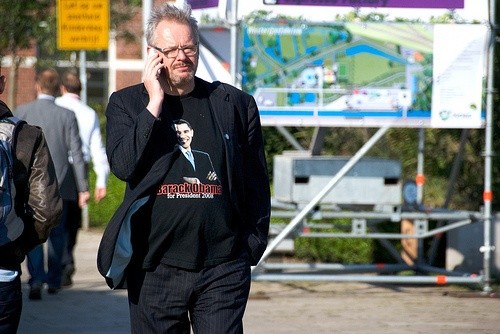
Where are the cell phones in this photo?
[149,48,162,74]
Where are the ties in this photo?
[186,151,195,172]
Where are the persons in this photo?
[0,66,111,334]
[96,6,271,334]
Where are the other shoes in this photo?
[63,262,76,286]
[29,286,42,299]
[48,288,56,294]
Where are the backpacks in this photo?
[0,116,27,246]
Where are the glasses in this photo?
[148,43,198,59]
